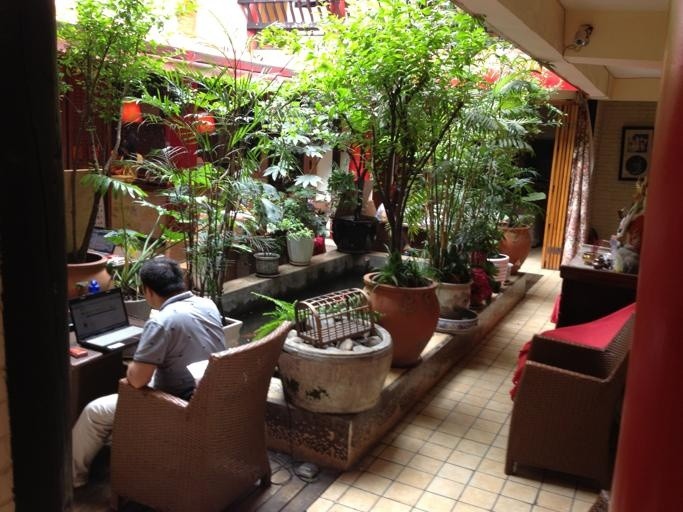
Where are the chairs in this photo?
[358,200,392,256]
[109,321,292,512]
[504,312,636,489]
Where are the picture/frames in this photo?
[618,124,653,180]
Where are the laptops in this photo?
[69,286,144,351]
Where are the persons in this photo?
[71,256,227,499]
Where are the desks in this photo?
[64,320,146,512]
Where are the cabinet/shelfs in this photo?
[555,243,638,327]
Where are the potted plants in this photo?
[249,290,393,414]
[124,6,307,349]
[54,0,199,307]
[159,190,276,296]
[500,71,567,274]
[307,17,379,255]
[486,51,532,291]
[253,200,280,278]
[453,225,505,268]
[250,0,499,368]
[286,227,314,265]
[437,304,478,335]
[422,51,558,315]
